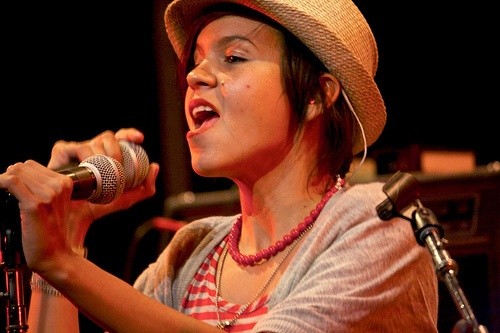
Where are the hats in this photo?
[164,0,386,153]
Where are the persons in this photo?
[0,0,439,333]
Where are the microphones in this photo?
[0,154,127,210]
[63,139,150,192]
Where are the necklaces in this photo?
[215,219,315,331]
[228,177,349,268]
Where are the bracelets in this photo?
[30,275,65,299]
